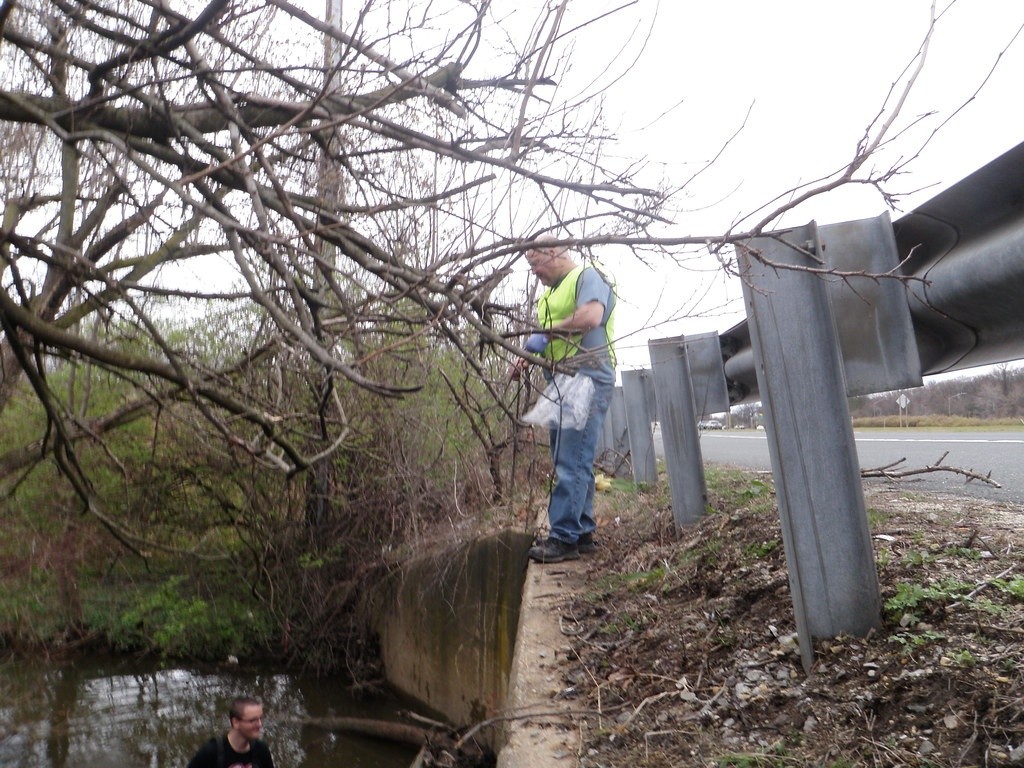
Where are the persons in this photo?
[505,236,618,563]
[185,697,275,768]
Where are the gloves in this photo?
[526,333,548,355]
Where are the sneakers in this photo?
[528,537,580,563]
[577,532,596,553]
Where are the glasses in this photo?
[240,714,263,724]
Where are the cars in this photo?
[735,425,745,429]
[706,420,722,430]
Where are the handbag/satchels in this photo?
[520,373,595,431]
